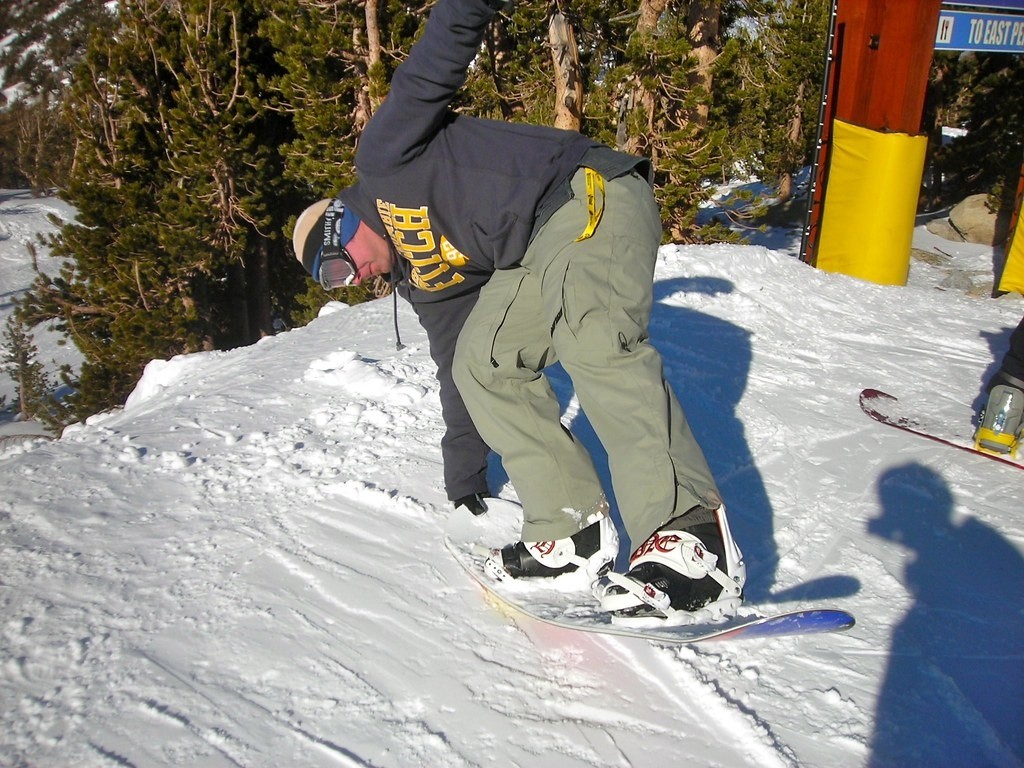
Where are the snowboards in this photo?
[860,388,1024,469]
[444,496,856,644]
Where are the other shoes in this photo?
[976,375,1024,453]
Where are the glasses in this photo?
[318,201,356,290]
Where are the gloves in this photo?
[452,492,488,515]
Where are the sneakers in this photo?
[607,505,743,620]
[486,516,616,584]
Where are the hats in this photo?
[294,198,359,283]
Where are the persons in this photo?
[293,0,748,619]
